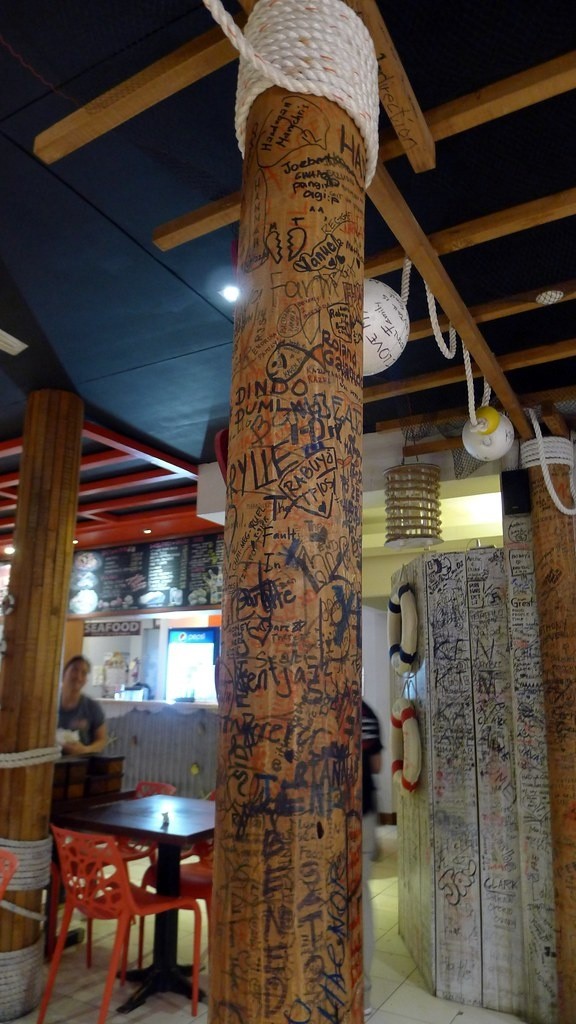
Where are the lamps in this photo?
[383,428,443,550]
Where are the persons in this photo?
[56,654,107,767]
[361,698,383,1016]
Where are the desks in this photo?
[80,795,215,1014]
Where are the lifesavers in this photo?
[385,584,418,674]
[388,697,422,793]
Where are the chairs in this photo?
[36,783,215,1024]
